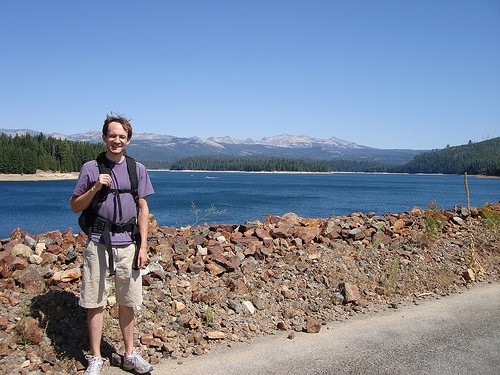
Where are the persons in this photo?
[69,112,155,375]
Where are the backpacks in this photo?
[78,152,142,239]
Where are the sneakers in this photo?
[82,355,106,375]
[124,351,153,374]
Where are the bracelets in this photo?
[139,244,148,249]
